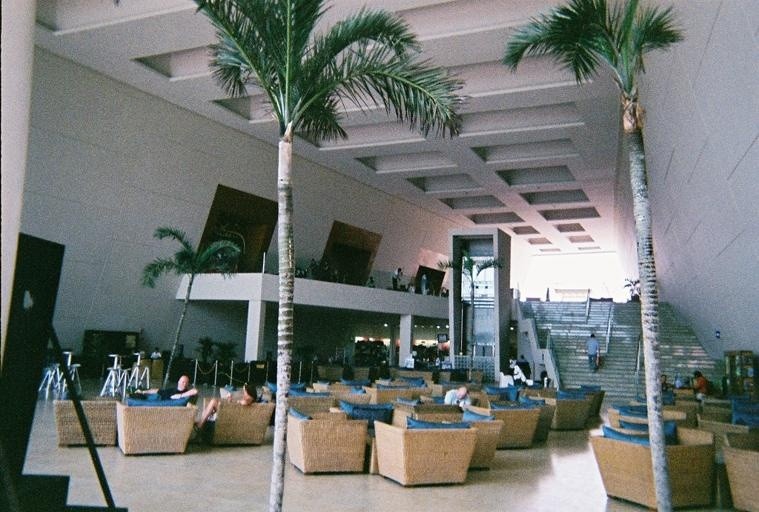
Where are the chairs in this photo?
[52,386,277,457]
[257,364,606,486]
[590,378,759,511]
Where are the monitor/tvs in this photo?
[438,333,448,343]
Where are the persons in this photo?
[343,359,354,382]
[431,366,441,384]
[584,334,600,372]
[661,374,670,391]
[134,374,198,400]
[444,384,470,408]
[150,348,161,360]
[369,361,380,386]
[195,384,258,432]
[498,355,531,388]
[380,361,390,380]
[296,259,449,297]
[691,371,708,394]
